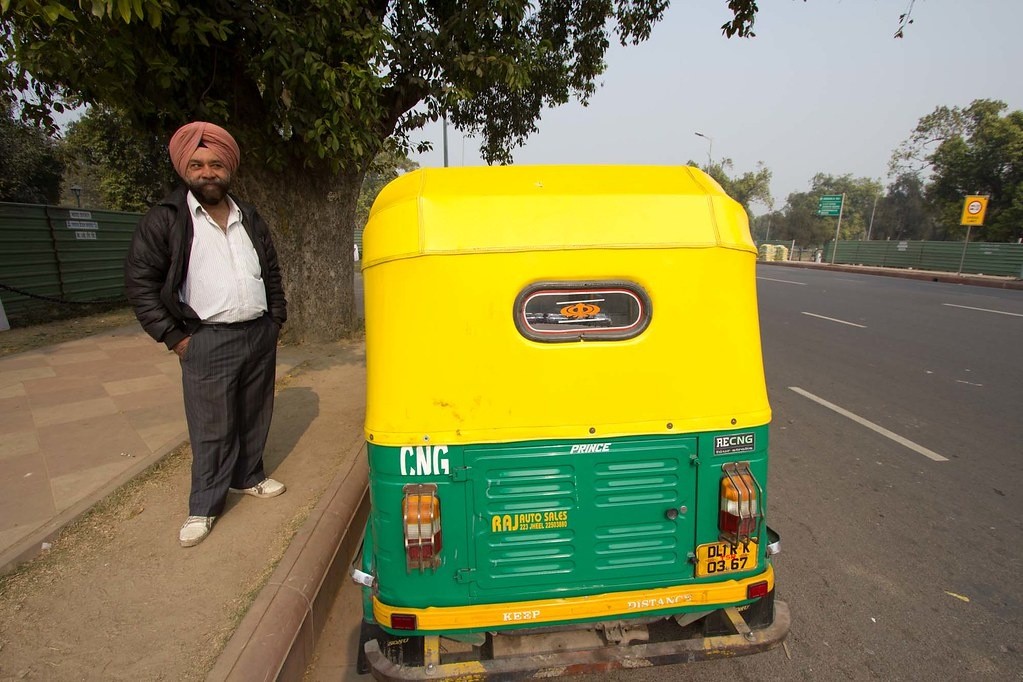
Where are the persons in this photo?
[124,121,288,548]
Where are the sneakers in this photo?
[228,476,286,499]
[178,514,216,547]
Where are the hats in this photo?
[168,122,241,181]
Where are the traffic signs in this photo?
[816,192,845,264]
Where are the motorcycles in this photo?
[351,164,792,682]
